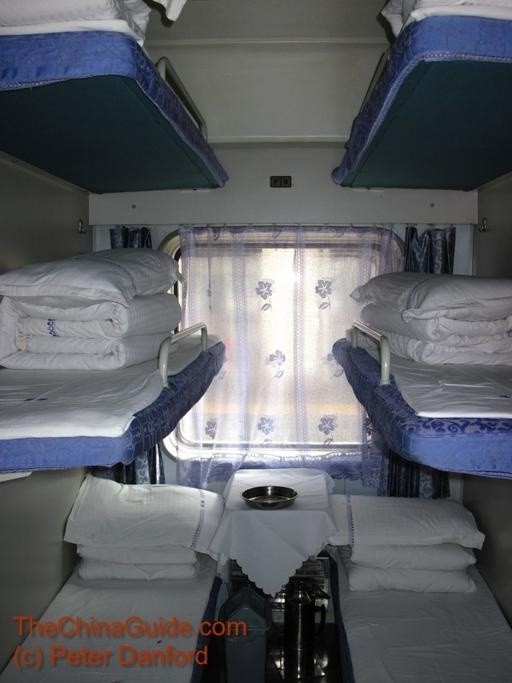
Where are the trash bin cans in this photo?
[218,587,272,683]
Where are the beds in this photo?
[2,29,229,196]
[330,547,512,682]
[330,16,511,191]
[0,322,226,479]
[0,560,224,683]
[333,320,512,481]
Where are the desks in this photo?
[224,468,333,561]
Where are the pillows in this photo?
[61,470,225,560]
[329,494,487,551]
[0,246,183,308]
[348,271,512,325]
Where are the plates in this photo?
[238,485,298,510]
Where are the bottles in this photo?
[284,575,329,652]
